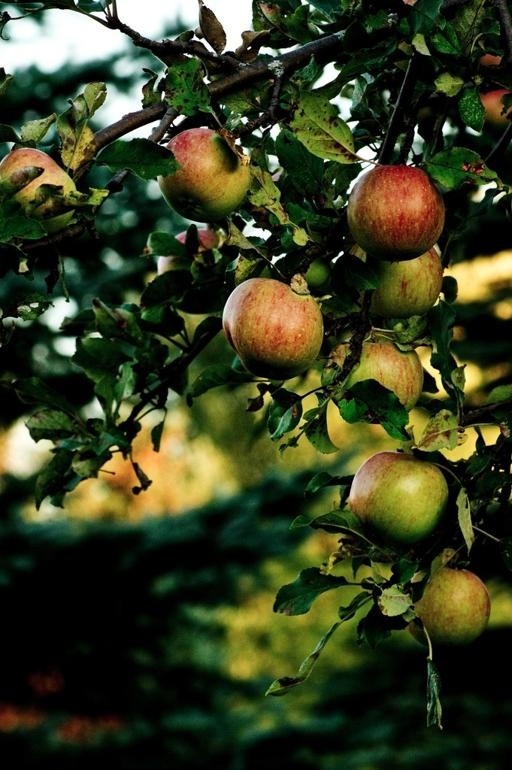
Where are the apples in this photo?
[0,148,77,216]
[408,566,491,649]
[222,277,325,380]
[346,165,446,262]
[349,451,449,546]
[477,89,512,132]
[349,243,443,318]
[157,128,251,222]
[325,328,424,424]
[305,259,333,290]
[480,54,502,67]
[157,228,219,277]
